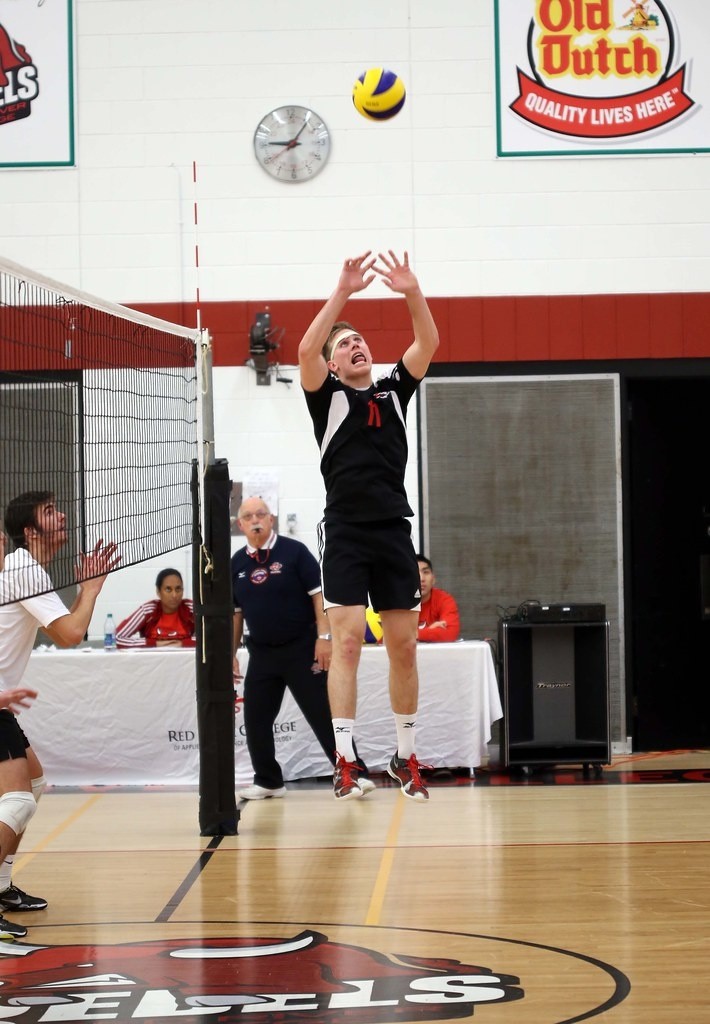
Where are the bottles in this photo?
[104,613,116,653]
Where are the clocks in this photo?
[253,106,331,183]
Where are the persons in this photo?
[419,555,462,641]
[0,491,122,942]
[233,496,378,800]
[299,250,442,802]
[113,567,196,647]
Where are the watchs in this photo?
[318,634,332,641]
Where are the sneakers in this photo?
[239,784,288,799]
[358,778,376,795]
[387,749,430,801]
[0,881,48,911]
[333,751,364,801]
[0,913,28,938]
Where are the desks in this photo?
[10,640,505,787]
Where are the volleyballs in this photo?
[350,66,408,122]
[363,606,384,645]
